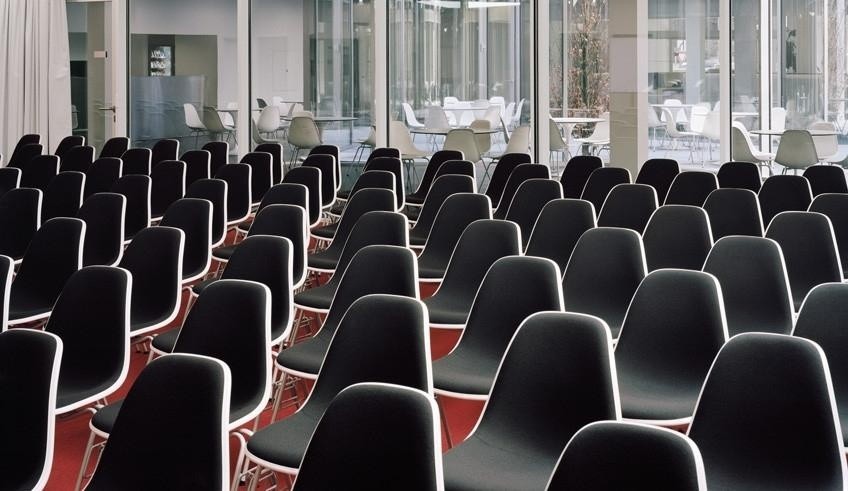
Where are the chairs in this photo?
[549,109,611,177]
[647,98,847,180]
[0,132,847,490]
[184,98,376,176]
[389,93,530,193]
[69,104,80,130]
